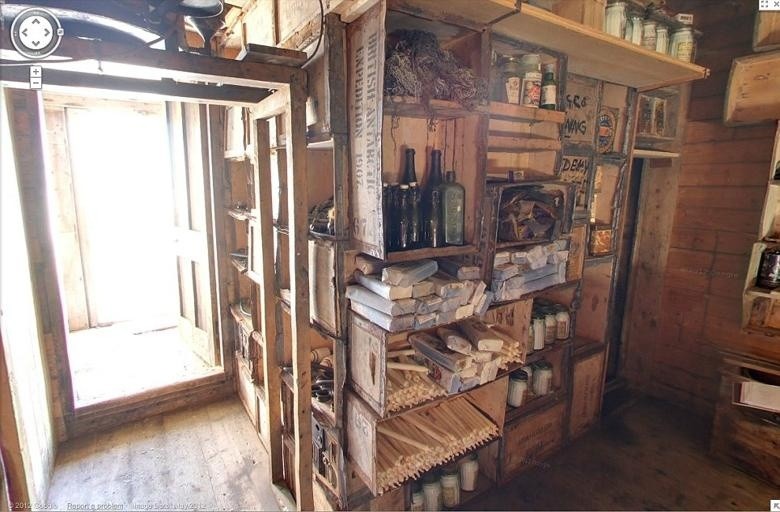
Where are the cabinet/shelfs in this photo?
[223,13,350,511]
[711,118,780,489]
[340,0,712,512]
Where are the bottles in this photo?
[383,182,389,233]
[423,150,445,247]
[668,25,694,62]
[626,16,634,42]
[532,314,546,351]
[556,304,569,340]
[402,150,418,185]
[395,185,410,249]
[424,474,441,512]
[533,361,554,396]
[499,56,522,105]
[392,183,399,203]
[441,466,460,509]
[542,308,557,344]
[459,453,480,492]
[508,370,528,408]
[629,12,643,44]
[641,20,656,51]
[409,183,422,244]
[606,2,628,39]
[520,366,532,390]
[542,65,557,110]
[533,305,540,314]
[410,484,426,512]
[537,297,554,305]
[439,171,465,246]
[656,24,670,54]
[527,322,535,357]
